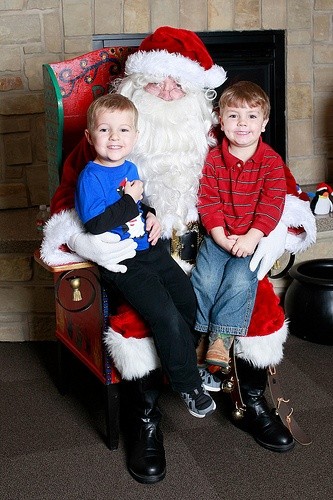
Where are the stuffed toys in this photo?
[310,181,333,215]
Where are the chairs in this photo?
[34,45,295,450]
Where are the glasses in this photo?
[145,88,186,100]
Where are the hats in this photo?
[125,25,228,88]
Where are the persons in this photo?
[75,93,224,419]
[40,25,317,485]
[190,81,287,369]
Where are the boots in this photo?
[231,356,295,452]
[120,368,167,484]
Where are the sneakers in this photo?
[196,364,223,392]
[180,385,216,419]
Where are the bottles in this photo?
[35,205,51,240]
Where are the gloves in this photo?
[66,232,138,273]
[249,220,288,281]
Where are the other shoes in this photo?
[195,333,234,368]
